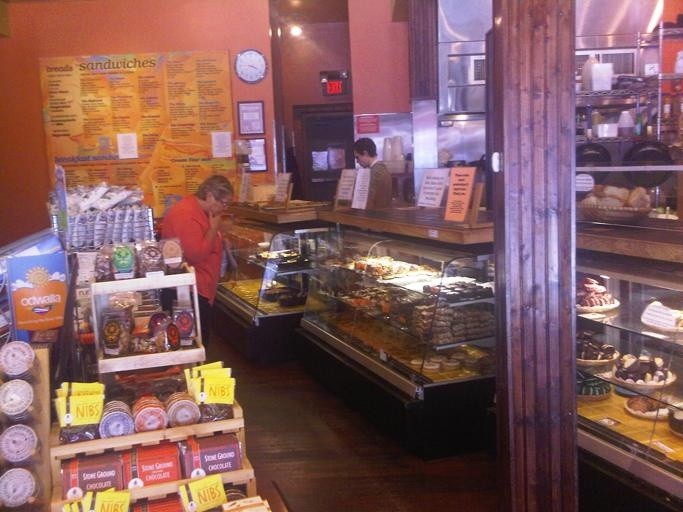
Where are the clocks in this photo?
[234,49,268,84]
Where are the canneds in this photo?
[0,340,41,511]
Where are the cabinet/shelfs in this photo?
[574,25,682,208]
[293,229,494,458]
[212,202,343,367]
[2,198,273,512]
[574,206,683,506]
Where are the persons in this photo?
[160,174,234,349]
[351,137,395,214]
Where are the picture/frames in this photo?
[242,138,268,172]
[237,100,265,134]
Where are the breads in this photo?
[575,331,682,432]
[641,301,682,333]
[576,274,616,311]
[578,181,653,224]
[306,253,496,378]
